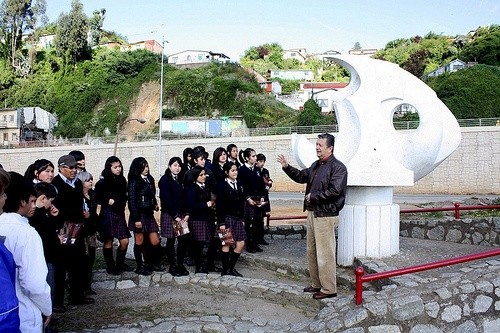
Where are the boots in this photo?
[132,241,165,275]
[117,246,134,271]
[221,251,243,276]
[103,247,122,274]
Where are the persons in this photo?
[277,132,348,299]
[0,143,274,333]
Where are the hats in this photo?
[57,154,82,166]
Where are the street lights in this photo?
[114,118,146,156]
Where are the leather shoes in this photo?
[85,289,97,294]
[70,296,95,303]
[304,286,321,292]
[246,244,263,252]
[53,305,67,312]
[186,256,221,273]
[257,238,269,244]
[313,291,337,298]
[65,279,70,290]
[170,263,189,275]
